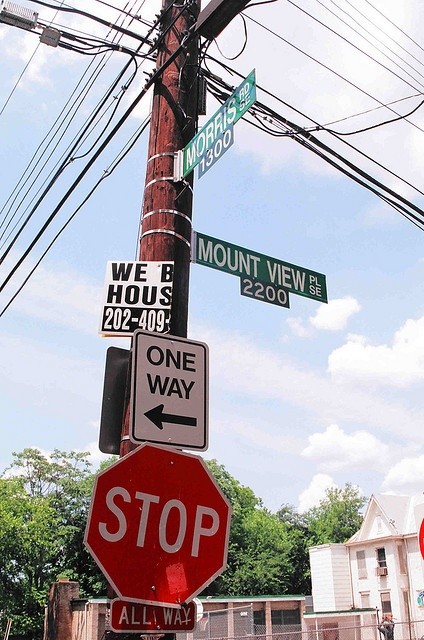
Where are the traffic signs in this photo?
[129,328,210,452]
[176,67,263,190]
[181,226,332,312]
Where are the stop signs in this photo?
[83,440,234,609]
